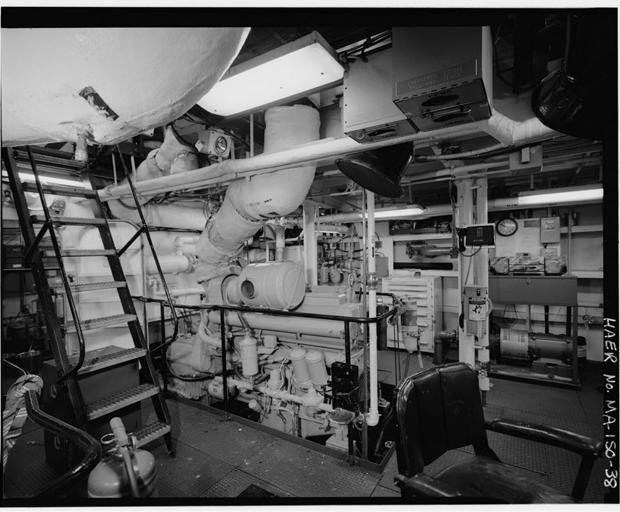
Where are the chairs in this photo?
[394,361,602,504]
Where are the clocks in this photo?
[496,217,517,237]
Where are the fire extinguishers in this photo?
[87,416,160,499]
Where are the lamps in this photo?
[359,204,425,219]
[197,31,346,121]
[517,184,605,206]
